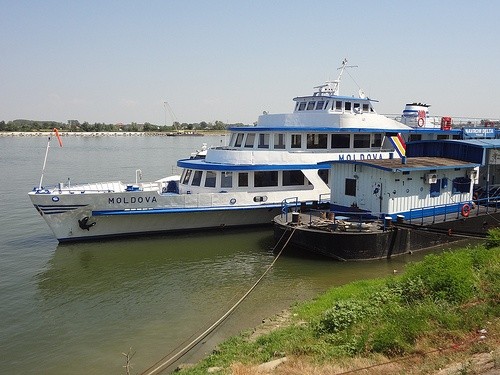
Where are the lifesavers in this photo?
[417,118,424,127]
[461,204,471,216]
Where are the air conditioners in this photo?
[425,173,437,184]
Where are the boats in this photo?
[270,156,500,262]
[27,58,414,245]
